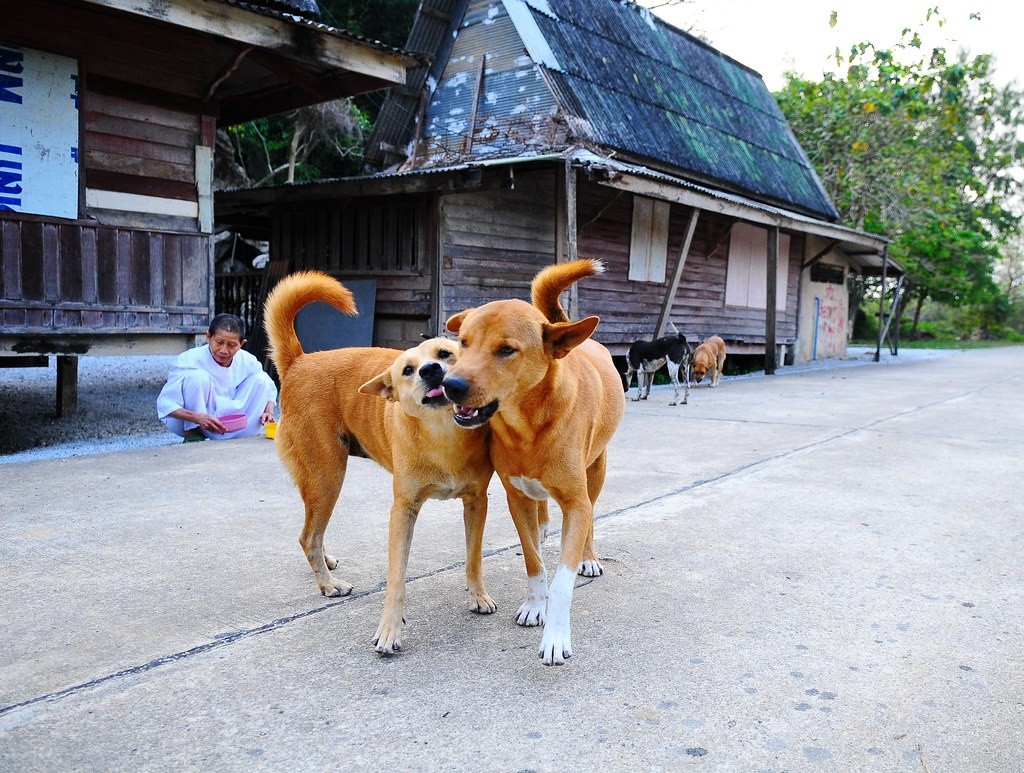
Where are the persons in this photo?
[156,313,278,444]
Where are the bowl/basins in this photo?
[265,423,278,439]
[217,414,246,432]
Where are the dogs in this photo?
[690,334,727,388]
[623,333,692,407]
[442,259,626,666]
[264,270,493,659]
